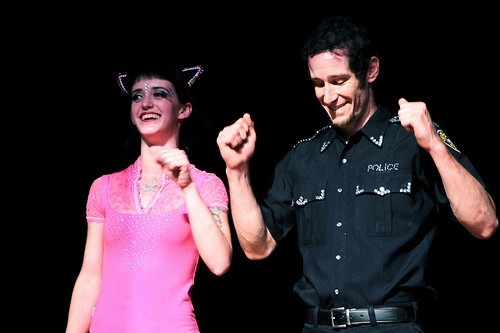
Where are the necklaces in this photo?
[140,182,159,193]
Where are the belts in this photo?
[304,302,422,328]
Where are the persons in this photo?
[216,25,499,333]
[65,56,232,333]
